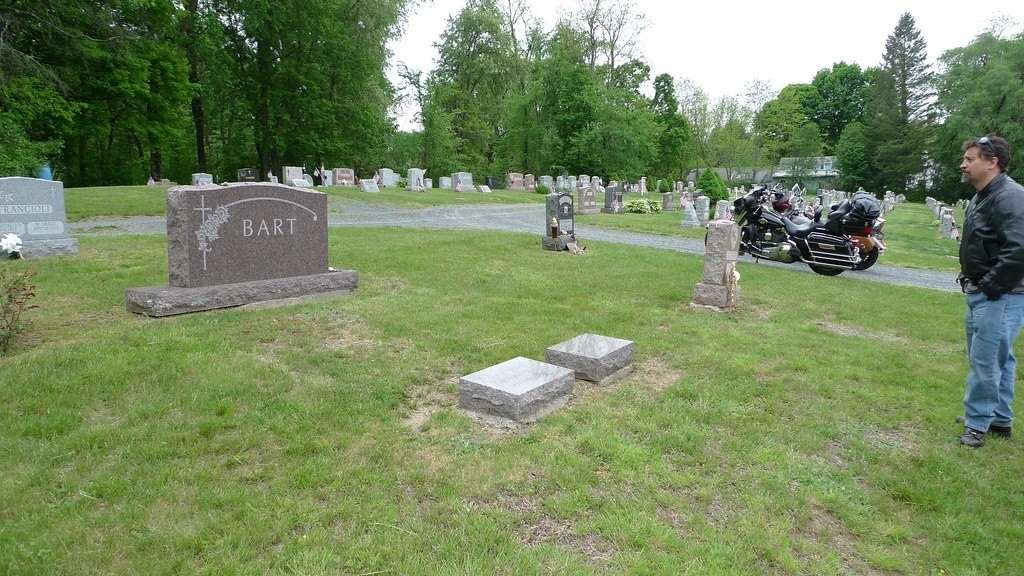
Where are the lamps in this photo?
[550,217,559,243]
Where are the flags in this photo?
[951,225,959,239]
[418,177,423,187]
[314,169,319,177]
[321,164,324,173]
[456,179,464,191]
[373,172,380,181]
[612,194,619,212]
[725,207,732,220]
[681,194,687,207]
[267,170,272,179]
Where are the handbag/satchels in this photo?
[849,191,881,222]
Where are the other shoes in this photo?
[960,428,985,448]
[956,415,1012,438]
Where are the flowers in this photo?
[0,232,23,259]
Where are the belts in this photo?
[961,277,971,284]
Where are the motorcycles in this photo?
[704,173,886,277]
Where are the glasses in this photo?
[979,137,997,154]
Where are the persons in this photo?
[955,136,1024,447]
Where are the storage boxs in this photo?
[839,217,874,237]
